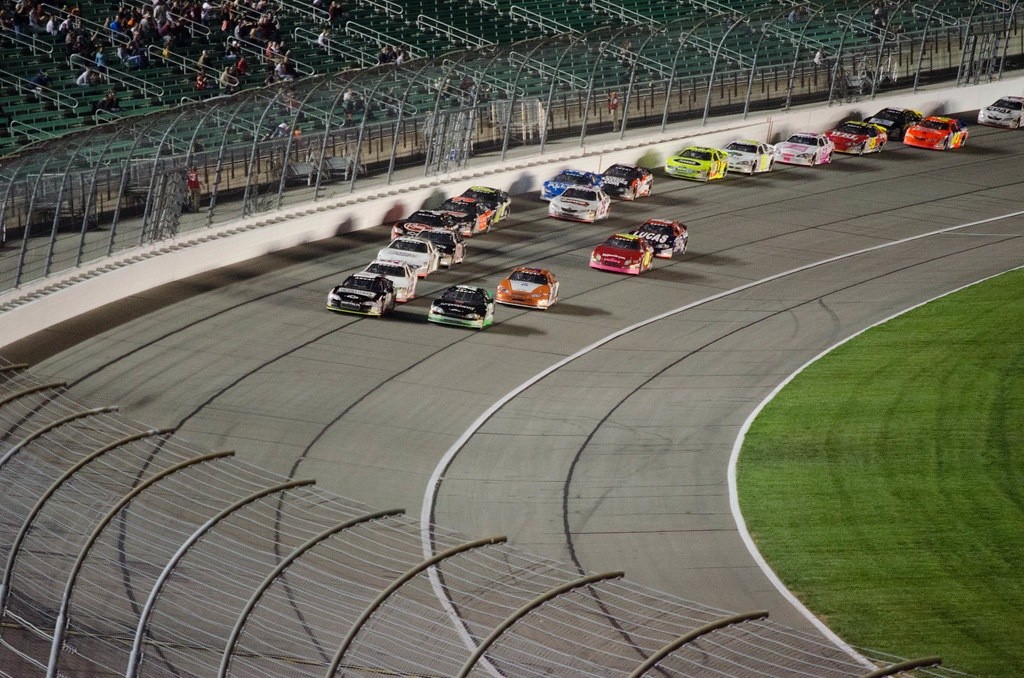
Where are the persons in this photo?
[607,90,620,132]
[868,0,892,43]
[265,116,278,136]
[276,119,290,137]
[94,89,123,115]
[294,124,305,143]
[191,136,205,152]
[788,5,808,25]
[328,42,503,122]
[618,38,664,91]
[720,12,746,33]
[813,44,830,68]
[188,165,205,213]
[375,281,383,292]
[0,0,356,101]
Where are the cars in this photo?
[774,132,835,168]
[722,138,776,175]
[664,146,728,182]
[978,96,1024,130]
[629,218,688,258]
[431,196,494,238]
[589,233,654,275]
[354,259,417,303]
[415,229,466,268]
[428,285,495,330]
[903,116,969,150]
[825,121,888,156]
[390,210,462,242]
[460,187,511,223]
[863,107,924,141]
[599,163,654,200]
[540,168,605,201]
[377,236,440,279]
[496,266,560,311]
[326,276,397,318]
[548,185,611,223]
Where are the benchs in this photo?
[0,0,1024,232]
